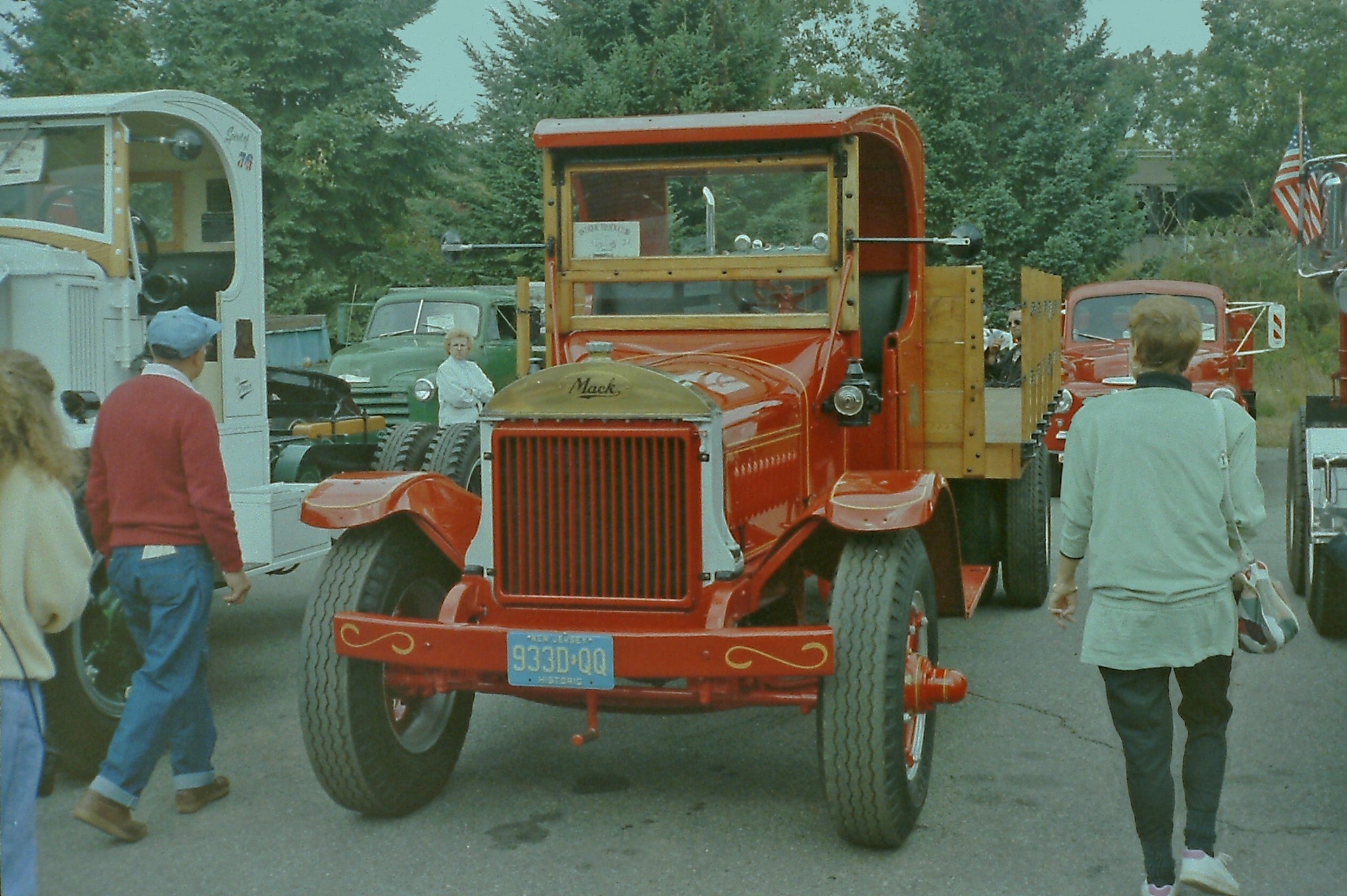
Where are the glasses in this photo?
[1008,319,1021,327]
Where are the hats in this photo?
[145,305,222,359]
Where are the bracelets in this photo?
[1052,584,1078,595]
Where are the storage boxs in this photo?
[228,480,332,566]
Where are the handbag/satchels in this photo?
[36,745,61,798]
[1231,560,1300,656]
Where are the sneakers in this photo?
[1140,878,1174,896]
[72,789,147,842]
[175,776,230,813]
[1178,846,1240,896]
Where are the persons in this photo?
[435,327,495,428]
[984,310,1021,388]
[71,306,252,842]
[0,346,94,896]
[1047,293,1270,895]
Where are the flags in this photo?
[1271,114,1329,249]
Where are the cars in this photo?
[1270,89,1347,638]
[1047,277,1287,499]
[294,99,1065,852]
[1,84,483,783]
[324,289,547,429]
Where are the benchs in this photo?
[587,260,909,403]
[129,252,239,334]
